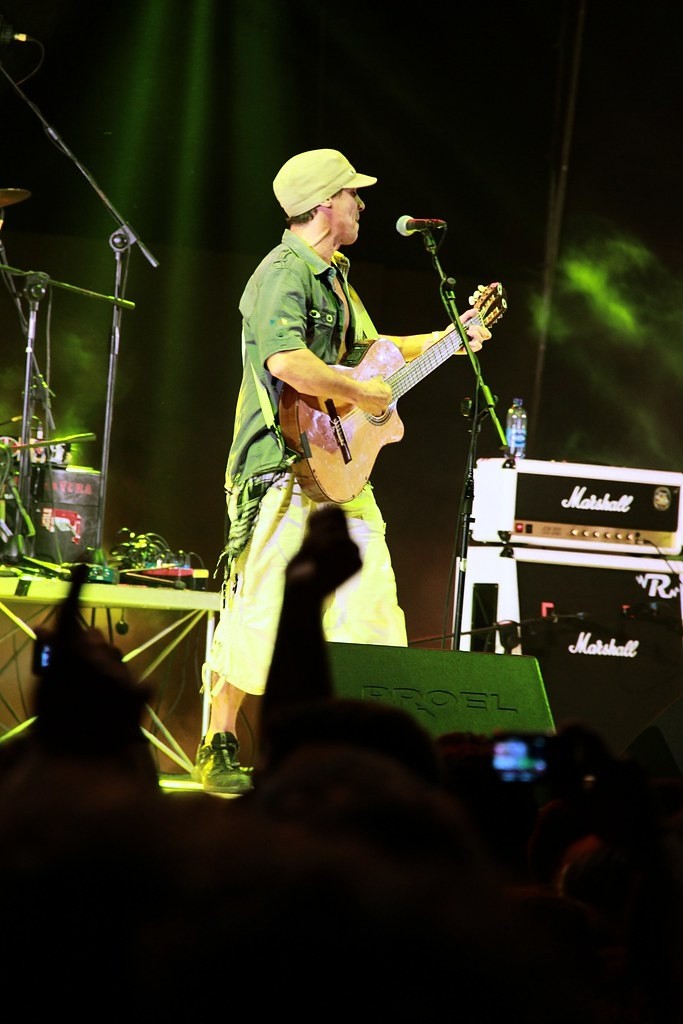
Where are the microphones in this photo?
[0,33,33,43]
[397,215,446,236]
[620,601,665,620]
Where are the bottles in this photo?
[504,398,527,458]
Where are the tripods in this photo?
[0,65,190,590]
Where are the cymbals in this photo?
[0,187,34,207]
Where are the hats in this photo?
[273,149,380,217]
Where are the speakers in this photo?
[325,545,683,781]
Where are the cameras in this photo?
[489,733,549,782]
[31,627,57,675]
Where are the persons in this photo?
[193,148,492,795]
[0,629,683,1024]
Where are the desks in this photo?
[0,574,224,786]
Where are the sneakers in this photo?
[193,731,251,792]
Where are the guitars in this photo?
[279,280,509,506]
